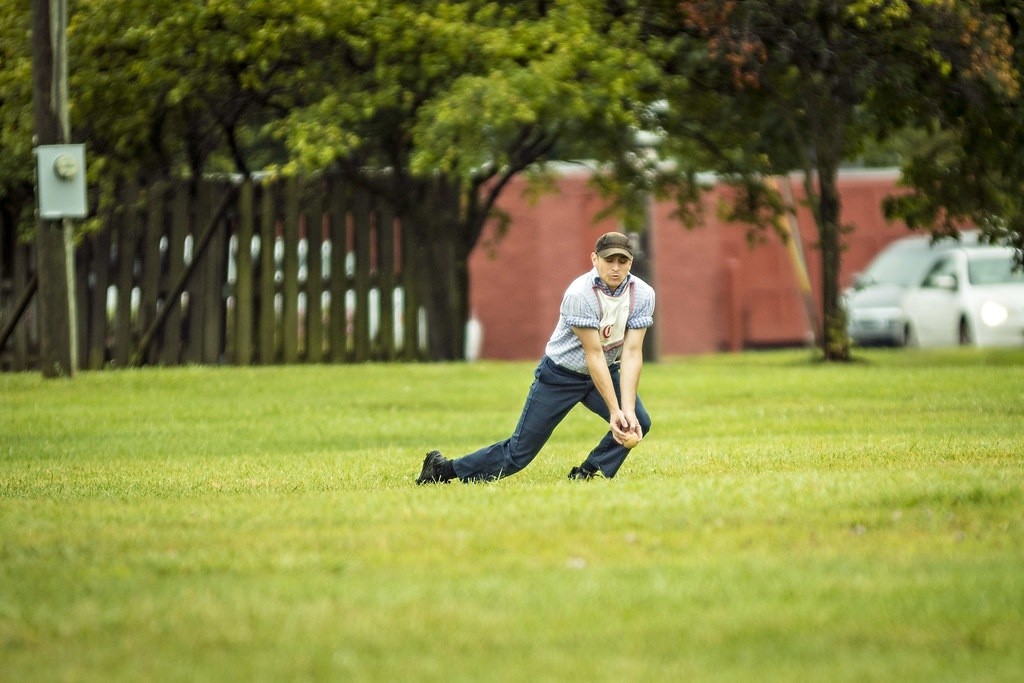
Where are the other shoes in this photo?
[567,466,596,484]
[414,450,452,485]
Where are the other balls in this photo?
[622,430,639,449]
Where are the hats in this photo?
[594,231,634,263]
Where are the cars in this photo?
[843,230,1024,347]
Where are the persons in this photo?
[416,231,655,488]
